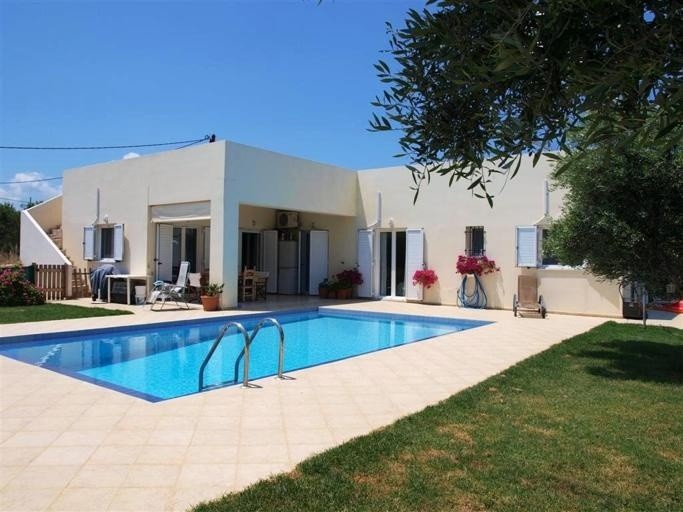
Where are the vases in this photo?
[347,288,353,298]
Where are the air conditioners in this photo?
[276,211,298,229]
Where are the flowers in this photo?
[337,269,363,289]
[455,255,500,276]
[413,269,438,289]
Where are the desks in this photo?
[239,271,269,301]
[106,274,153,305]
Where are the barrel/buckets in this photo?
[135,294,145,305]
[134,286,146,294]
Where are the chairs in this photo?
[512,275,545,318]
[240,265,257,302]
[150,261,191,311]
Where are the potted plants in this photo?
[200,283,225,311]
[319,279,347,298]
